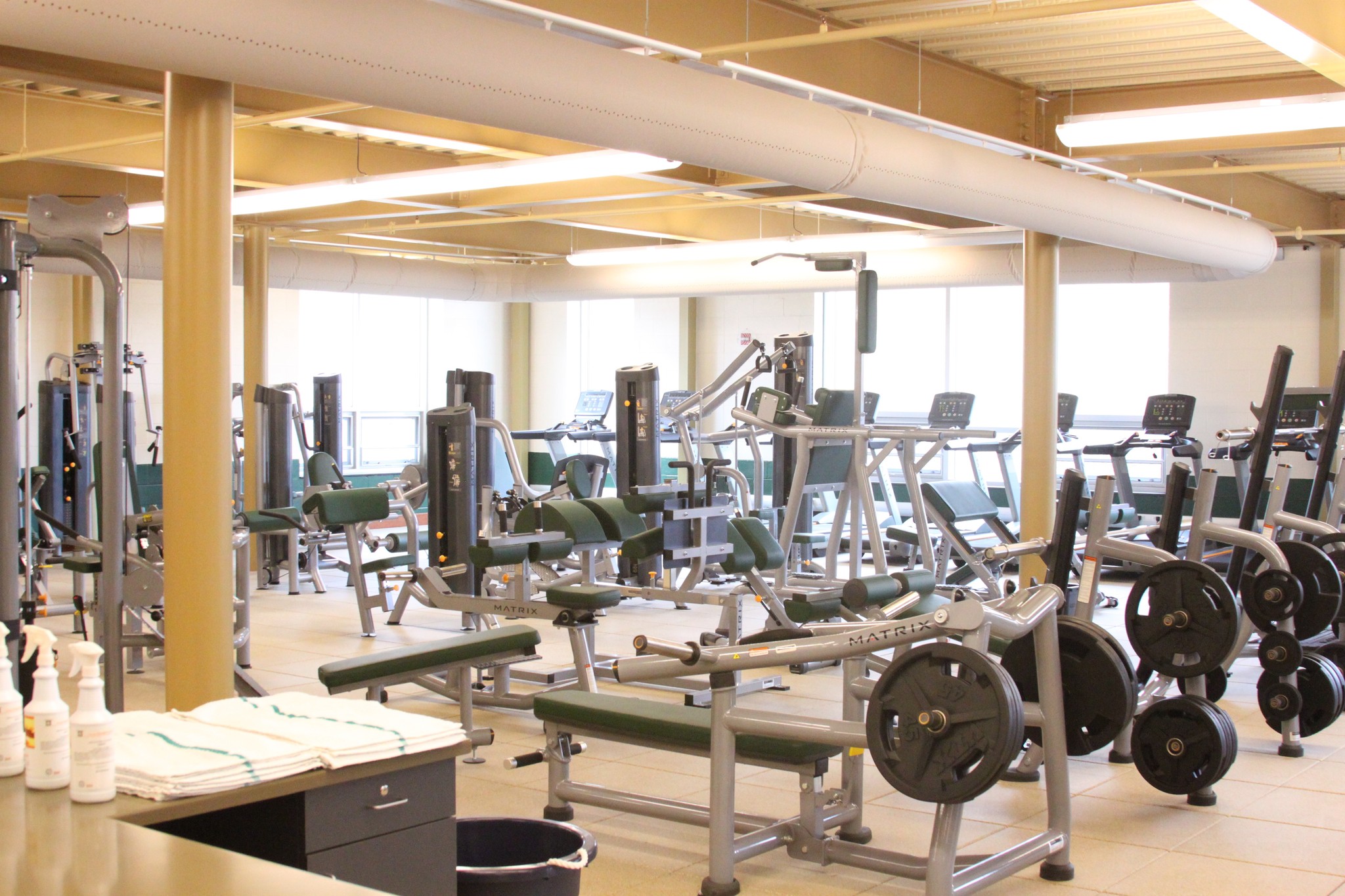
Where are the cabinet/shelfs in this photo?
[301,757,459,896]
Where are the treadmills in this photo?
[543,389,1333,572]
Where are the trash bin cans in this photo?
[456,817,598,896]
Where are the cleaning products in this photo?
[67,640,116,805]
[18,625,70,792]
[0,621,27,779]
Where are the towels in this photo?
[109,690,468,803]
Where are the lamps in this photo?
[1052,8,1345,151]
[127,103,943,272]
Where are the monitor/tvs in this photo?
[1142,394,1195,431]
[574,389,614,419]
[660,390,695,408]
[927,392,975,426]
[1276,410,1319,429]
[1057,393,1078,428]
